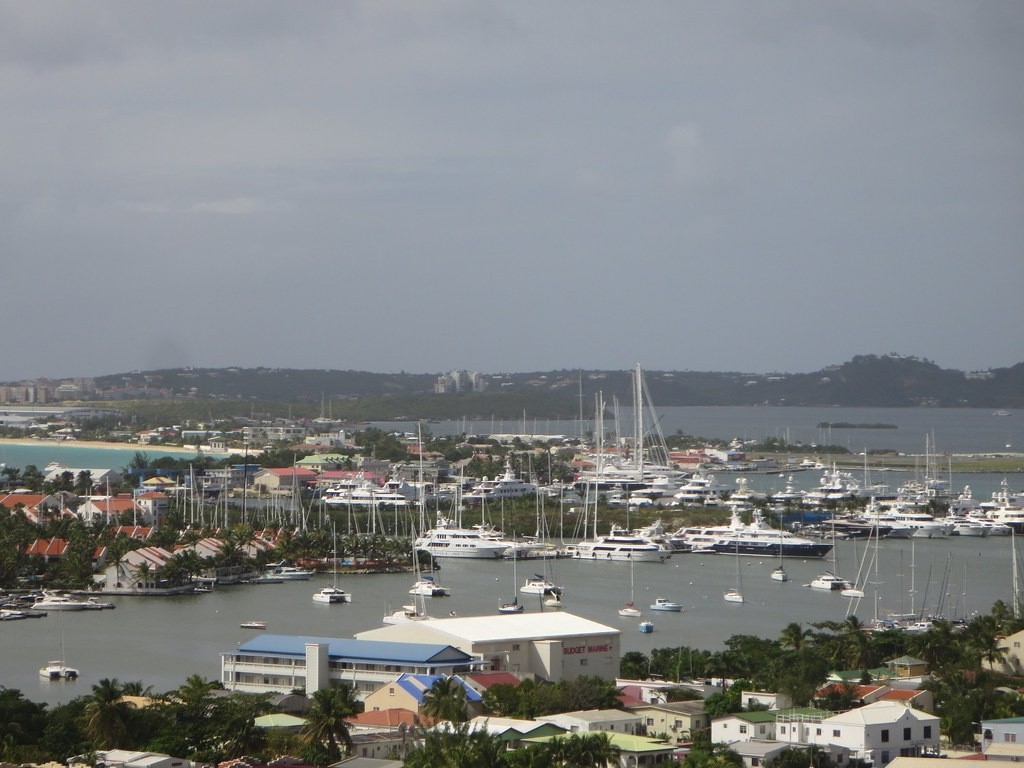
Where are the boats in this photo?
[194,586,212,593]
[641,623,654,633]
[0,588,116,620]
[240,621,267,628]
[243,562,314,583]
[545,598,562,608]
[651,598,683,612]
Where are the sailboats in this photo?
[39,596,79,679]
[312,519,352,604]
[320,360,1024,615]
